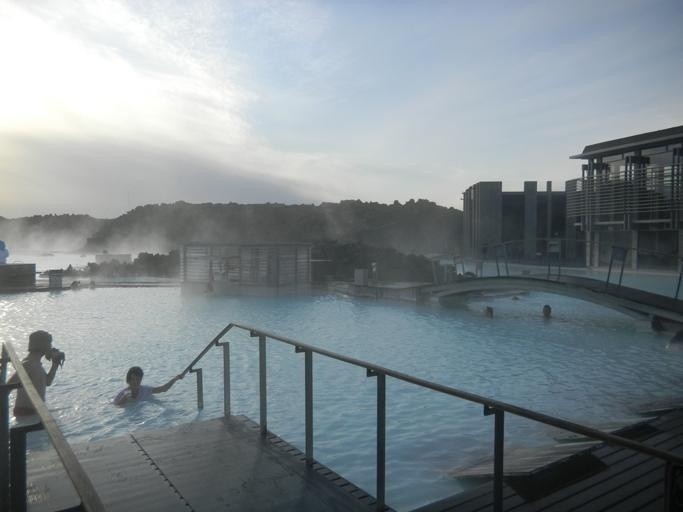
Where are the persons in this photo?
[483,306,493,317]
[112,366,181,405]
[542,305,552,316]
[7,329,61,417]
